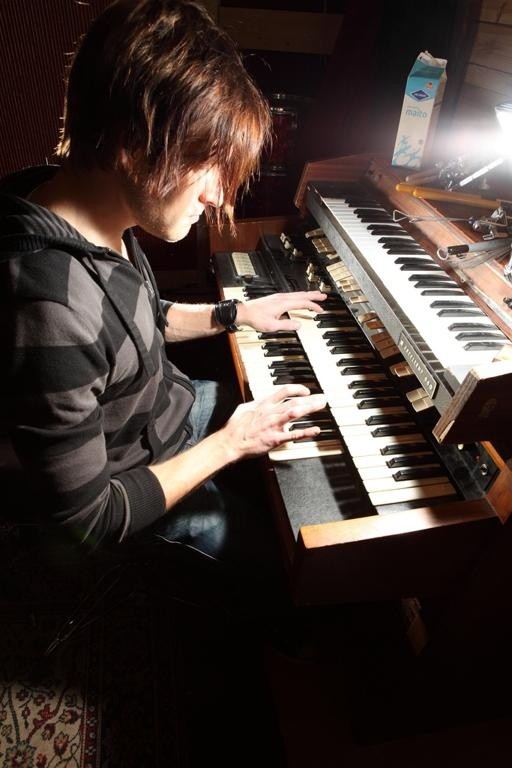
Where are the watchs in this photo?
[214,297,240,333]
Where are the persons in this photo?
[0,3,452,745]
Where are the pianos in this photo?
[207,216,512,555]
[293,150,512,446]
